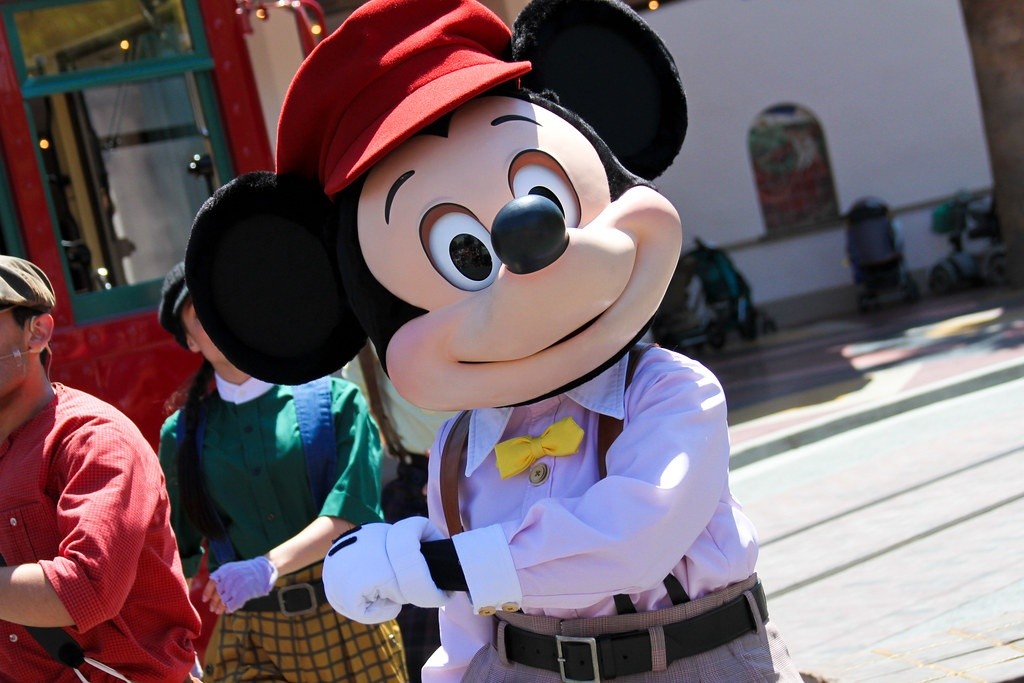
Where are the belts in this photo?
[238,578,329,617]
[491,585,768,683]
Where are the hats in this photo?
[158,260,189,333]
[0,254,57,312]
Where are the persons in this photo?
[0,256,202,683]
[158,260,462,682]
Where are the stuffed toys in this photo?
[186,1,779,682]
